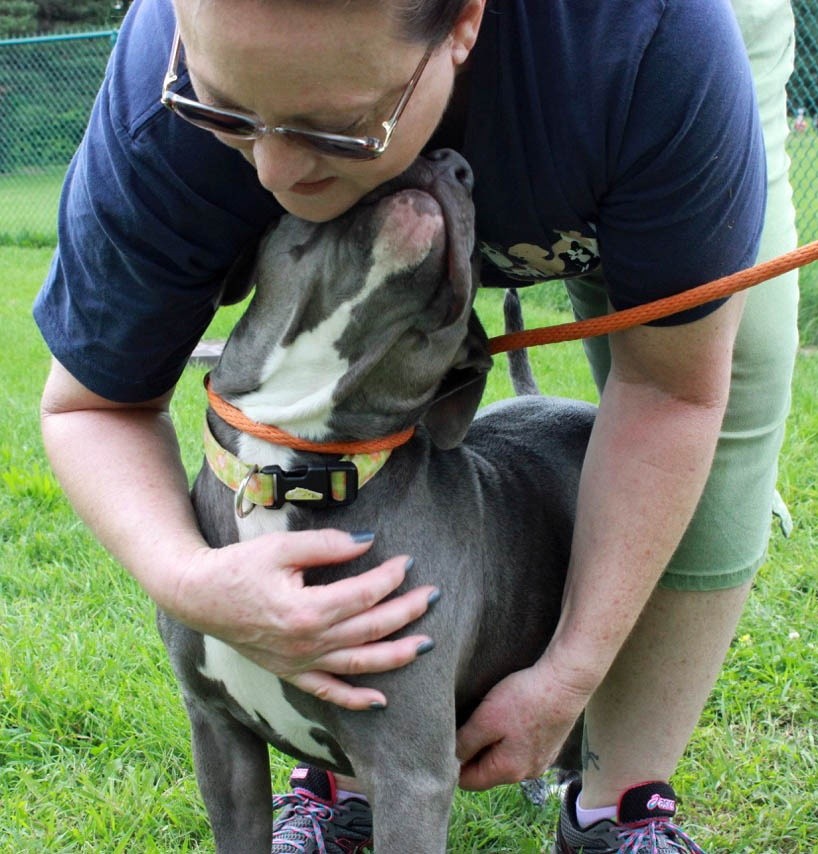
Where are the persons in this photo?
[31,1,802,854]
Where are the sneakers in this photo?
[552,777,706,854]
[271,760,374,854]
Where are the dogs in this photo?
[157,145,599,854]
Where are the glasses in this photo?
[161,18,443,158]
